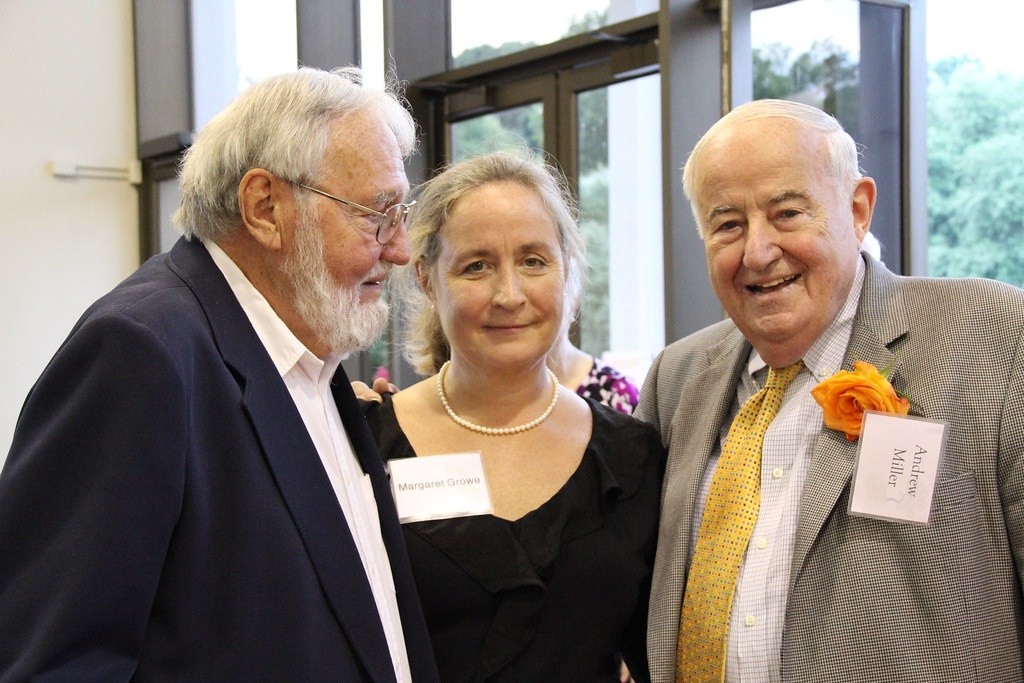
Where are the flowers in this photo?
[811,354,926,446]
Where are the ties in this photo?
[675,364,803,683]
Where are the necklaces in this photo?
[437,360,560,436]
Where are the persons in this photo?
[351,99,1024,683]
[543,318,645,415]
[356,153,666,683]
[0,67,437,683]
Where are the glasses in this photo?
[299,185,418,245]
